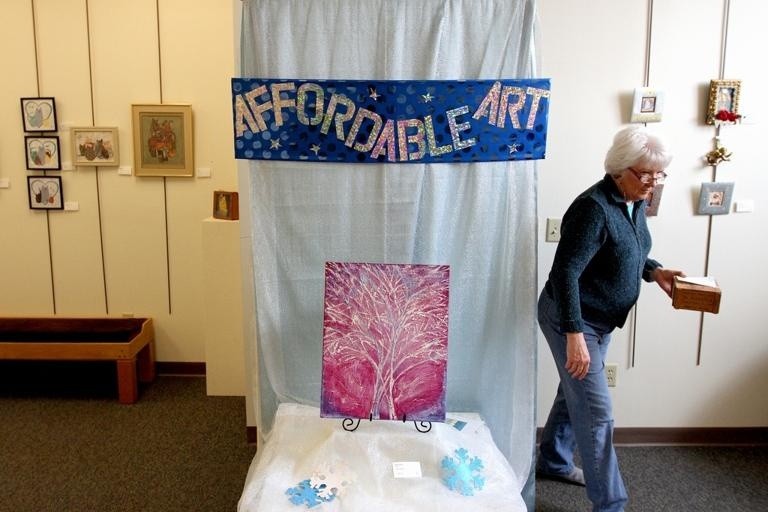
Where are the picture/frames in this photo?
[130,105,195,178]
[25,136,61,170]
[646,185,664,217]
[22,97,57,133]
[707,79,742,125]
[28,175,63,210]
[698,182,732,215]
[632,86,664,123]
[72,128,120,168]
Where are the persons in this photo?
[536,123,687,512]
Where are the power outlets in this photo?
[605,364,614,387]
[547,219,561,242]
[212,191,238,222]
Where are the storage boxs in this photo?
[672,272,721,314]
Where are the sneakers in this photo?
[537,461,585,485]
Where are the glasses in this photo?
[630,166,667,184]
